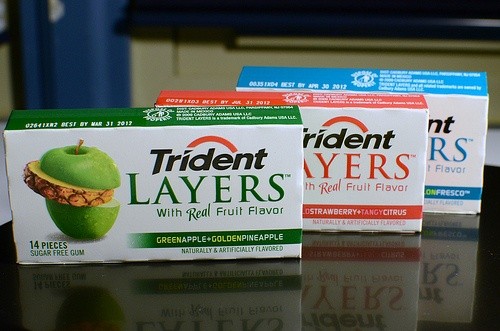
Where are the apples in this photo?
[45,195,120,240]
[38,139,121,189]
[52,284,127,328]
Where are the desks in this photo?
[0,163,500,331]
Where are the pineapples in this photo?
[21,160,114,207]
